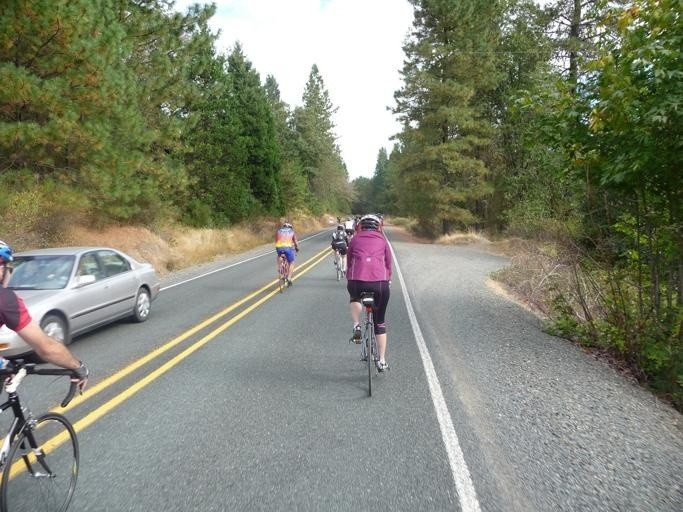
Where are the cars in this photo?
[0,244,160,360]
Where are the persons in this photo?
[346,214,393,372]
[1,241,89,395]
[345,212,384,246]
[275,223,299,285]
[332,225,350,275]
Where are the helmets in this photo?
[283,223,292,229]
[0,239,14,263]
[357,214,381,228]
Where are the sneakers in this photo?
[377,361,390,372]
[351,324,362,342]
[286,278,292,286]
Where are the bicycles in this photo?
[335,248,346,283]
[277,248,298,293]
[349,291,390,398]
[0,358,89,512]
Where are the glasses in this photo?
[1,266,13,273]
